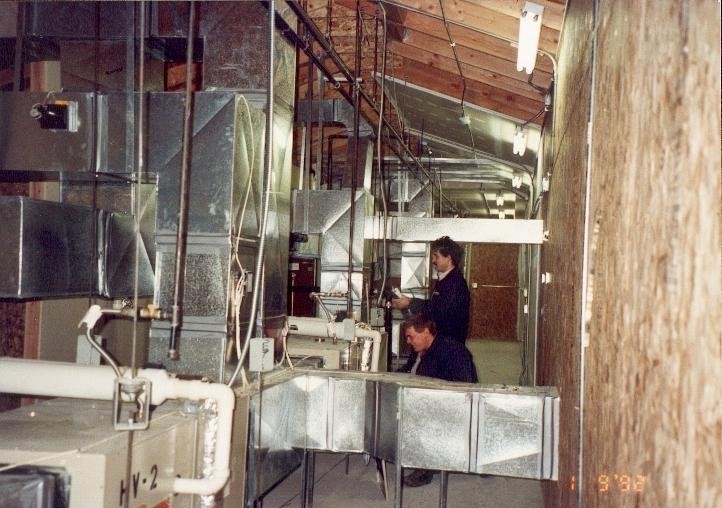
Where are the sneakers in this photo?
[403,469,433,487]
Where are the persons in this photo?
[394,315,478,488]
[389,237,471,346]
